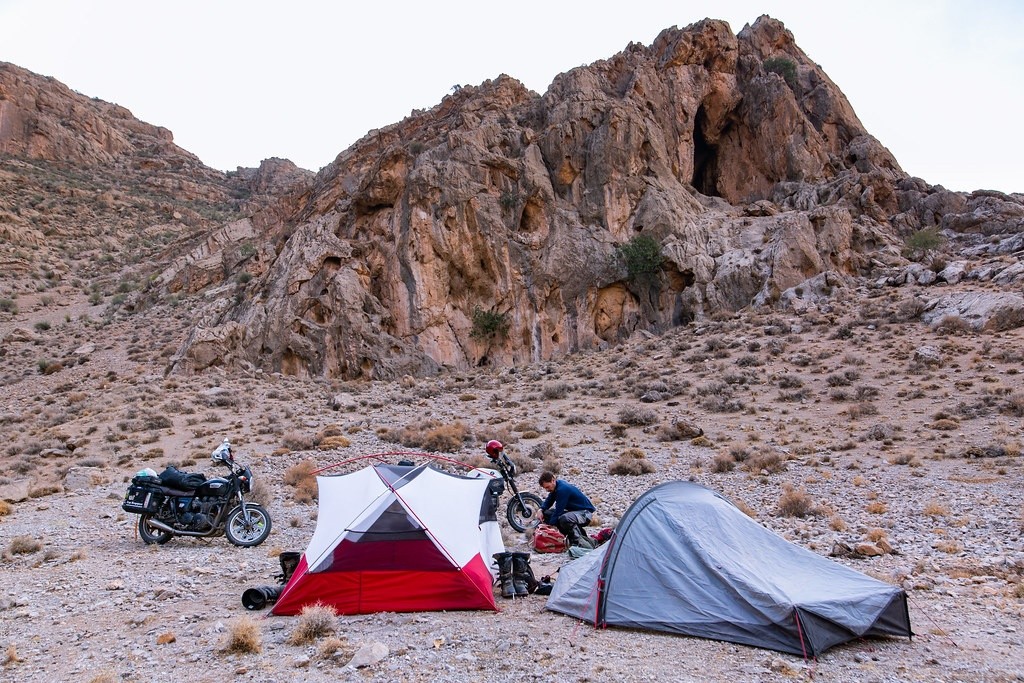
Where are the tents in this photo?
[269,465,499,612]
[545,480,914,659]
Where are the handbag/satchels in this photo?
[528,524,569,553]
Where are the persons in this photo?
[536,473,596,547]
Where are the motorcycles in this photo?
[121,438,272,548]
[397,440,544,533]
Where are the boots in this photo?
[274,552,300,585]
[493,552,517,596]
[512,552,530,595]
[242,584,286,610]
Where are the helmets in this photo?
[486,440,503,459]
[211,437,234,466]
